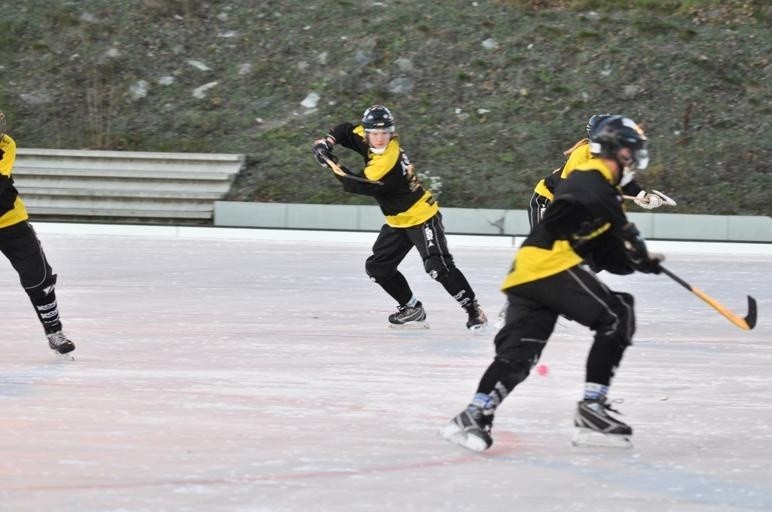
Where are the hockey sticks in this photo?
[657,263,758,331]
[623,189,676,206]
[319,150,384,186]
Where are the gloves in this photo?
[312,133,338,168]
[634,190,675,210]
[630,257,661,275]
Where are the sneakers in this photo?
[462,299,487,328]
[575,400,632,435]
[47,330,75,354]
[389,301,426,323]
[455,404,493,447]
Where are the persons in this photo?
[450,116,661,450]
[496,113,661,330]
[0,112,74,352]
[314,105,488,332]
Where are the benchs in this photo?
[0,143,247,225]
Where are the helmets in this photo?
[587,114,648,170]
[362,105,394,133]
